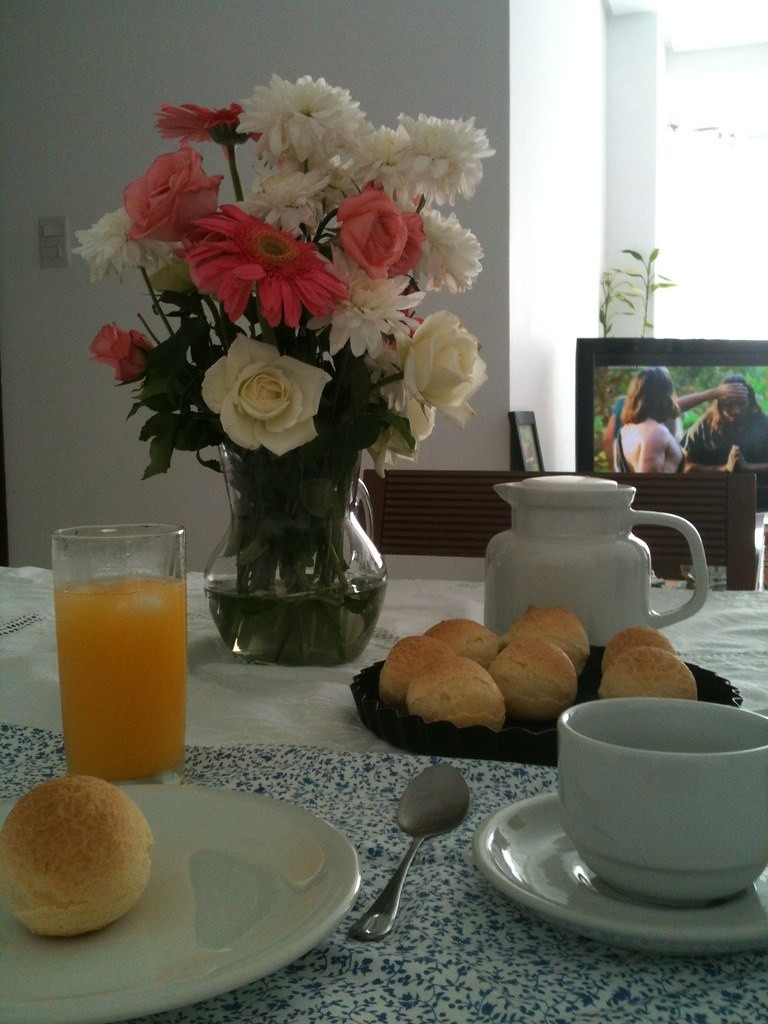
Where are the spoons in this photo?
[346,763,471,942]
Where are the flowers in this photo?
[72,72,493,663]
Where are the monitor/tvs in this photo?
[576,339,768,513]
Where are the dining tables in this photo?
[0,559,768,1024]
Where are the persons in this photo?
[613,367,748,474]
[679,375,768,507]
[604,396,626,468]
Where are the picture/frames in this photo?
[507,410,545,472]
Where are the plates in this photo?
[472,792,768,955]
[349,646,745,768]
[0,784,362,1024]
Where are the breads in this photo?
[379,604,698,730]
[0,776,154,936]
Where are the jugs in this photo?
[483,474,709,649]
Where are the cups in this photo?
[50,522,188,786]
[555,697,767,908]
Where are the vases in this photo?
[202,441,389,671]
